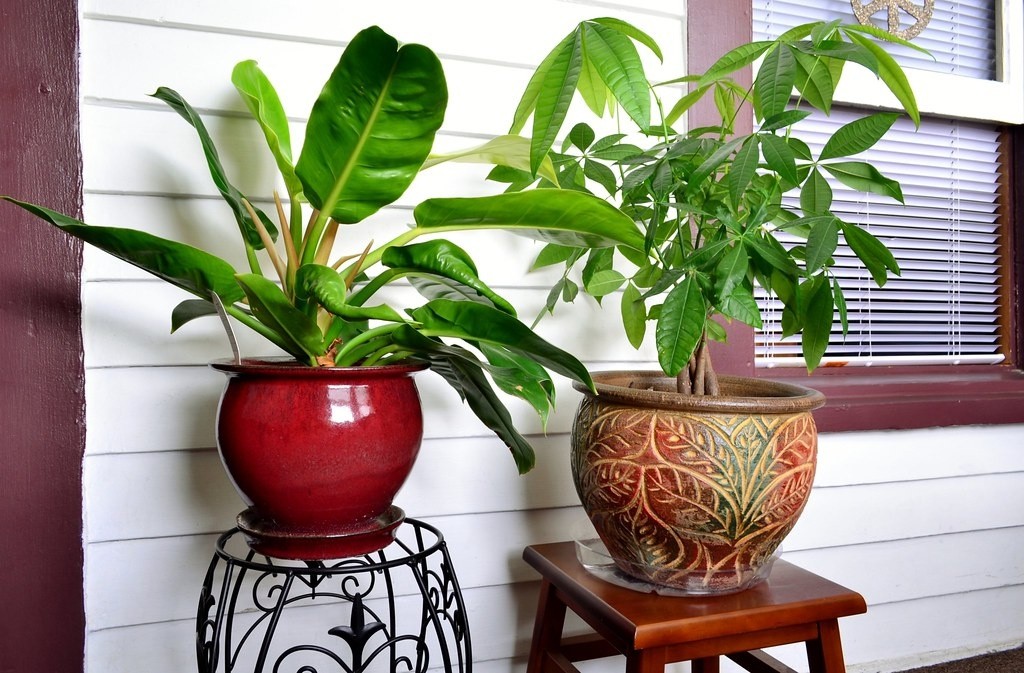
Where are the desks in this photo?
[521,539,866,673]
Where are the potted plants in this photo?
[485,17,937,594]
[0,23,600,562]
